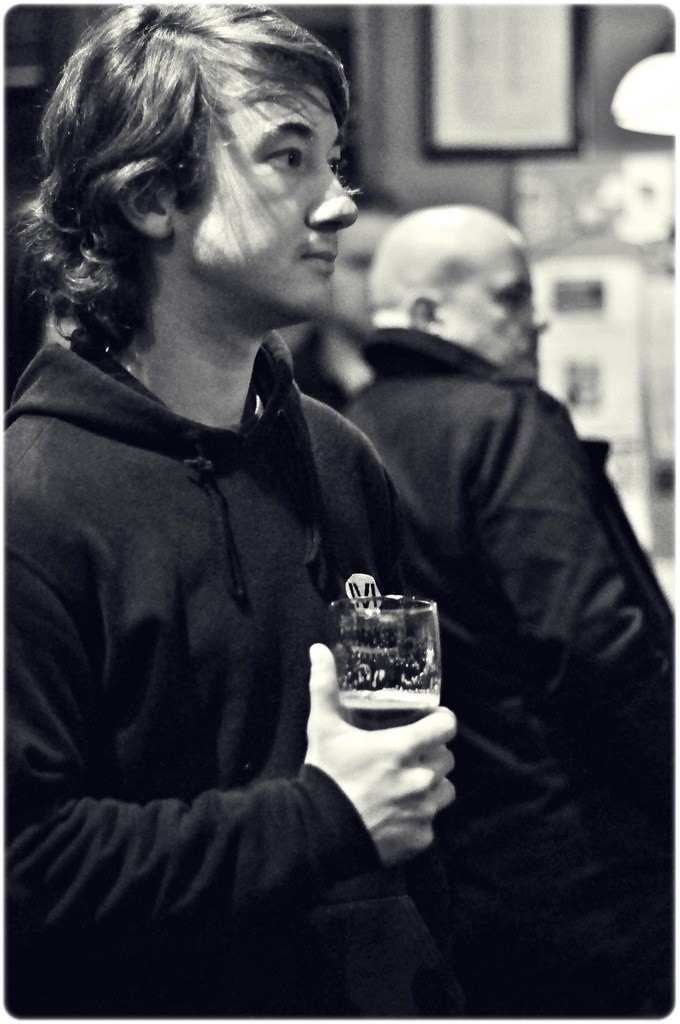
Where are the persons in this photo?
[336,202,676,789]
[3,4,676,1020]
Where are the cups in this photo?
[330,596,441,764]
